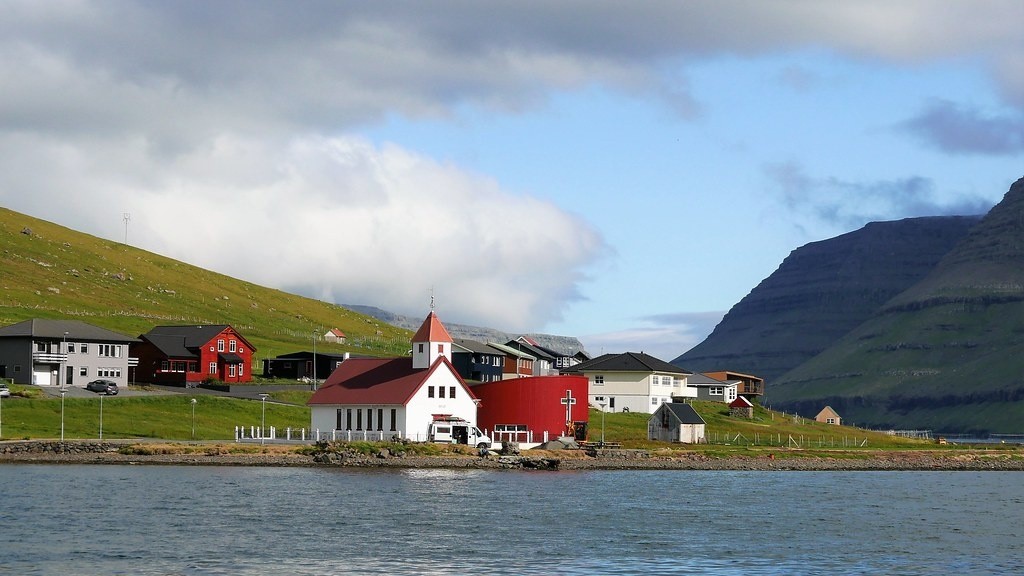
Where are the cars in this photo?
[0,384,10,398]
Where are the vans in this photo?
[428,421,491,450]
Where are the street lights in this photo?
[599,403,607,448]
[313,329,318,391]
[98,391,106,439]
[190,398,199,436]
[259,393,269,445]
[63,331,69,389]
[58,388,70,439]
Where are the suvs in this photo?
[87,379,119,394]
